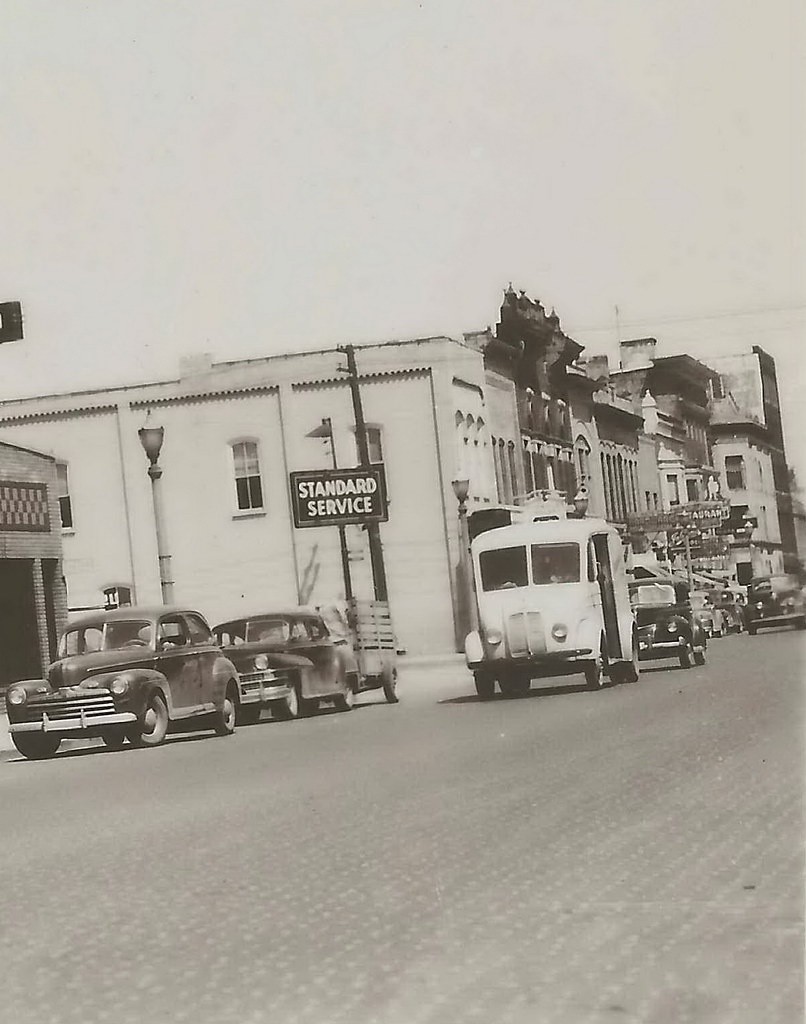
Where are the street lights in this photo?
[306,416,352,602]
[449,471,480,634]
[138,408,176,612]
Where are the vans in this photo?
[461,490,647,699]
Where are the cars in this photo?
[5,609,247,767]
[205,608,358,721]
[739,573,806,636]
[629,574,745,664]
